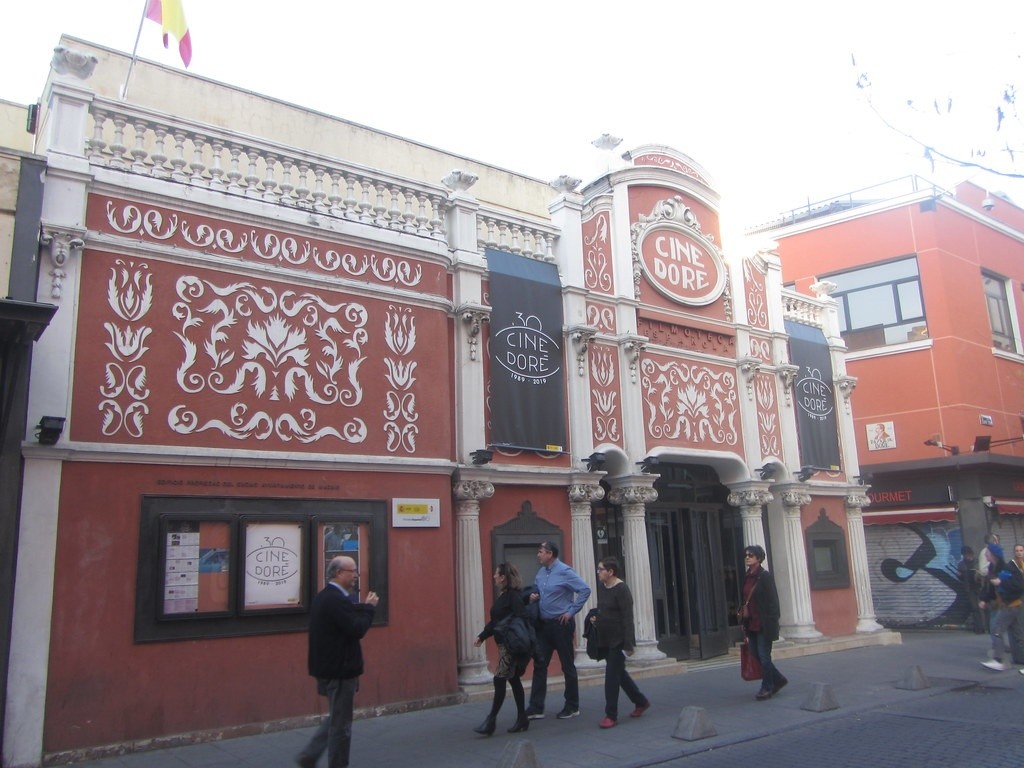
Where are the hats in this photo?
[987,543,1004,559]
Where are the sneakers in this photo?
[556,708,580,719]
[526,708,545,719]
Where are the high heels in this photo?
[507,711,529,733]
[473,714,496,735]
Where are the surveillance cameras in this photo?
[982,199,995,211]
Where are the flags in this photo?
[145,0,192,69]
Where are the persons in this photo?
[957,534,1024,675]
[324,524,358,550]
[589,557,652,727]
[740,545,787,699]
[295,556,380,768]
[525,542,591,718]
[471,562,530,735]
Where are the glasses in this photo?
[341,569,358,574]
[597,567,606,570]
[744,553,755,558]
[539,543,551,551]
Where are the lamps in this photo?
[640,455,660,473]
[33,416,68,445]
[585,453,606,473]
[794,468,815,484]
[924,439,958,455]
[854,472,874,485]
[471,448,494,465]
[757,462,776,479]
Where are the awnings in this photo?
[993,498,1024,515]
[862,508,956,526]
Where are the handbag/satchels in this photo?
[737,605,749,624]
[740,637,763,681]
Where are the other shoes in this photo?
[599,717,617,728]
[980,659,1004,671]
[296,752,315,768]
[1019,669,1023,674]
[756,685,774,699]
[772,676,787,694]
[631,703,650,716]
